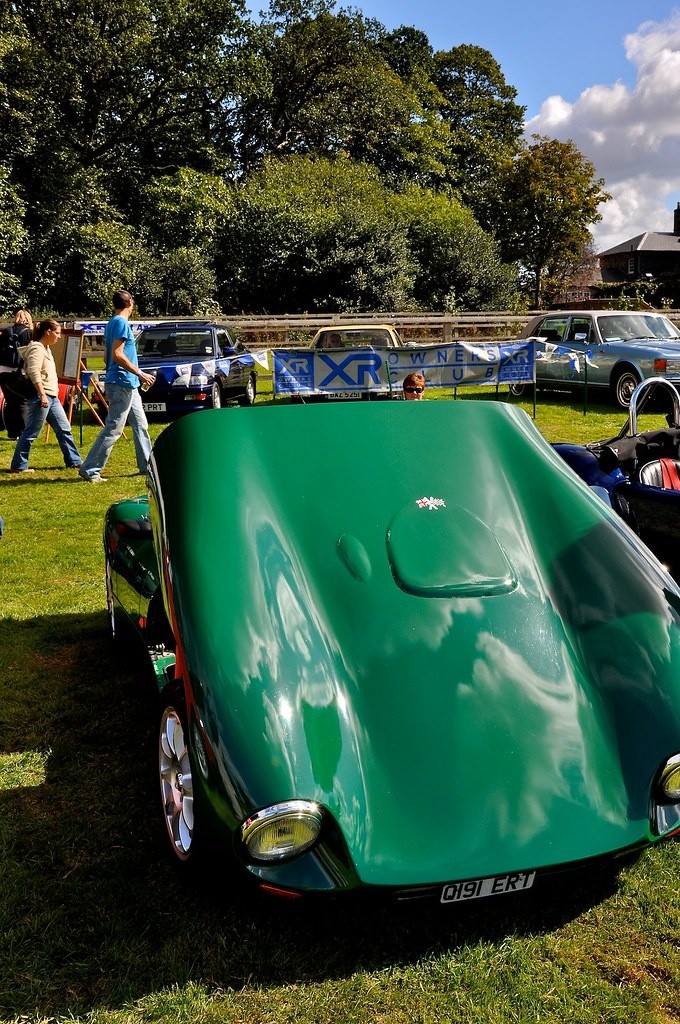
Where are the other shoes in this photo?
[69,460,84,469]
[11,466,34,472]
[7,436,19,440]
[79,470,108,483]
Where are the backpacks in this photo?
[0,326,30,368]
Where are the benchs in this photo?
[536,328,561,341]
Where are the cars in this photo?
[291,325,405,400]
[134,319,260,414]
[99,376,680,910]
[505,308,680,410]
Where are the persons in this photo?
[79,290,155,483]
[403,373,426,401]
[0,310,34,440]
[9,318,84,473]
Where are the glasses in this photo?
[405,387,424,393]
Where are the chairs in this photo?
[639,459,680,490]
[570,322,594,341]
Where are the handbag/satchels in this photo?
[600,427,680,483]
[609,483,680,563]
[4,359,37,399]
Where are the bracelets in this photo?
[136,369,142,376]
